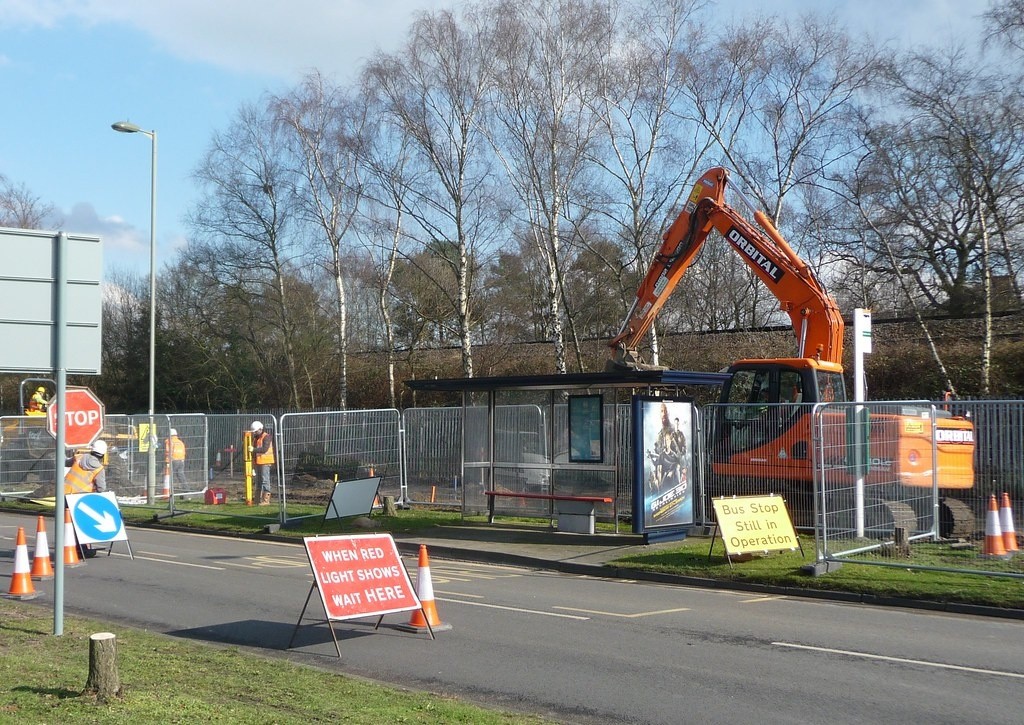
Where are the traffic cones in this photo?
[368,468,383,509]
[162,464,171,501]
[976,493,1016,561]
[213,450,222,468]
[49,508,84,568]
[998,491,1022,554]
[395,544,454,635]
[0,526,48,601]
[141,475,147,497]
[29,515,55,582]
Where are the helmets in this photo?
[251,420,263,432]
[92,440,107,456]
[38,387,45,393]
[170,429,178,436]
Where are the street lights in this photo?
[111,121,157,507]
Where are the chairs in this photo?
[787,393,802,419]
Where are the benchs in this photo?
[485,492,614,533]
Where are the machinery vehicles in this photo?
[0,377,138,482]
[598,166,976,540]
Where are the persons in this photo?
[247,420,275,505]
[64,440,107,558]
[654,404,686,492]
[29,386,51,412]
[165,429,193,500]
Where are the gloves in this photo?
[248,446,254,452]
[252,469,256,477]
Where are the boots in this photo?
[259,492,271,505]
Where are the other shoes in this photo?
[78,546,96,559]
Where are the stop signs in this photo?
[47,385,106,449]
